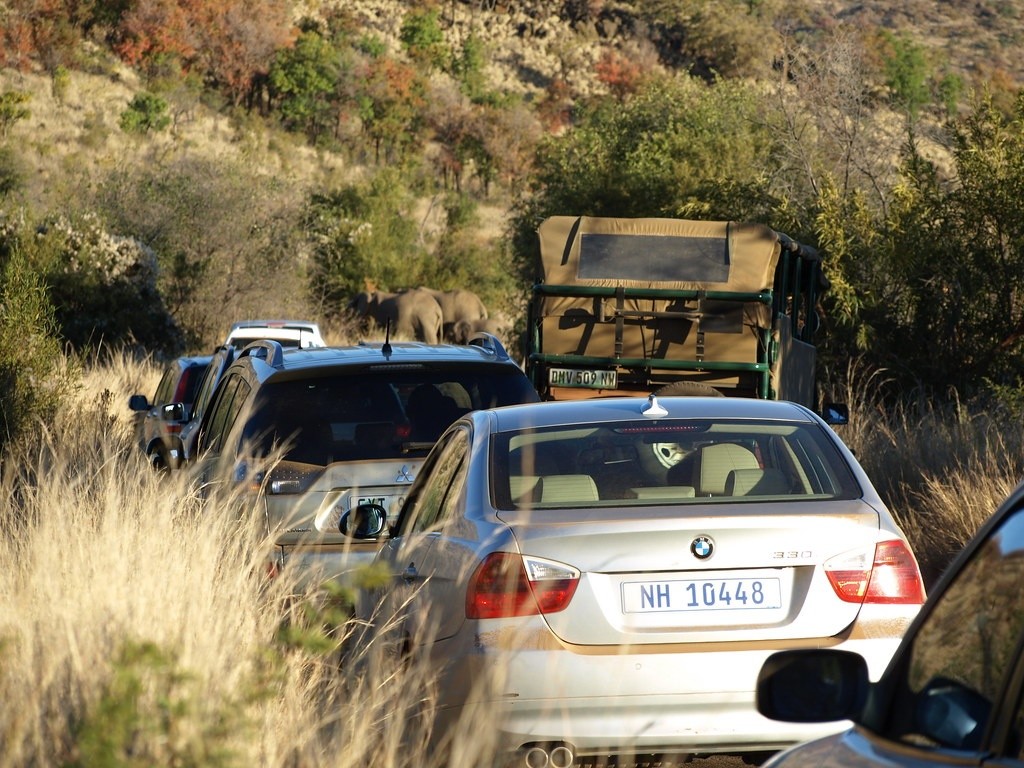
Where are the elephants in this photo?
[366,286,510,346]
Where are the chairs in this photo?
[508,443,790,504]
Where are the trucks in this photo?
[518,210,831,417]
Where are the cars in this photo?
[338,395,931,768]
[753,475,1024,768]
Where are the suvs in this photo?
[128,320,556,633]
